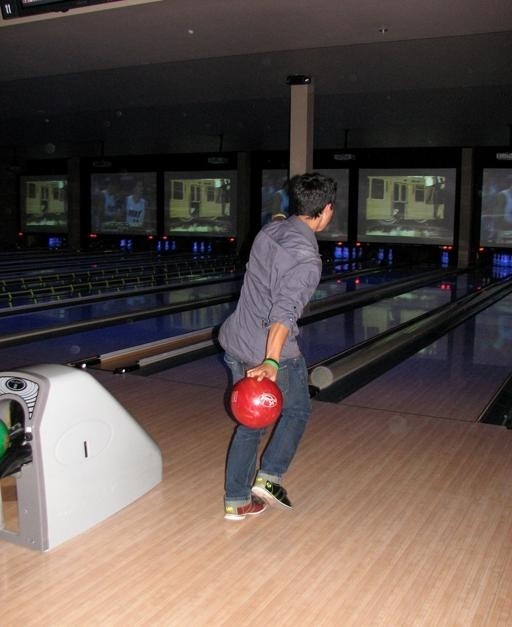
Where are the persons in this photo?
[121,180,151,228]
[216,169,339,521]
[492,177,512,236]
[96,176,123,229]
[271,178,290,214]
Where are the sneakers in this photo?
[223,501,267,521]
[252,474,293,511]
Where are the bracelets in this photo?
[272,213,287,220]
[260,358,280,370]
